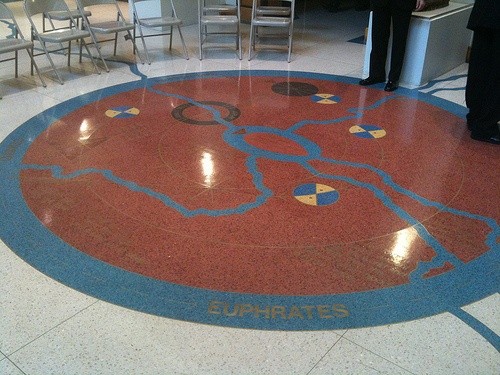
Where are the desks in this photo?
[364,0,475,86]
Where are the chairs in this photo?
[0,0,295,100]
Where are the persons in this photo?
[359,0,424,92]
[466,0,500,143]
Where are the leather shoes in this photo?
[359,74,384,86]
[475,123,500,144]
[384,81,398,92]
